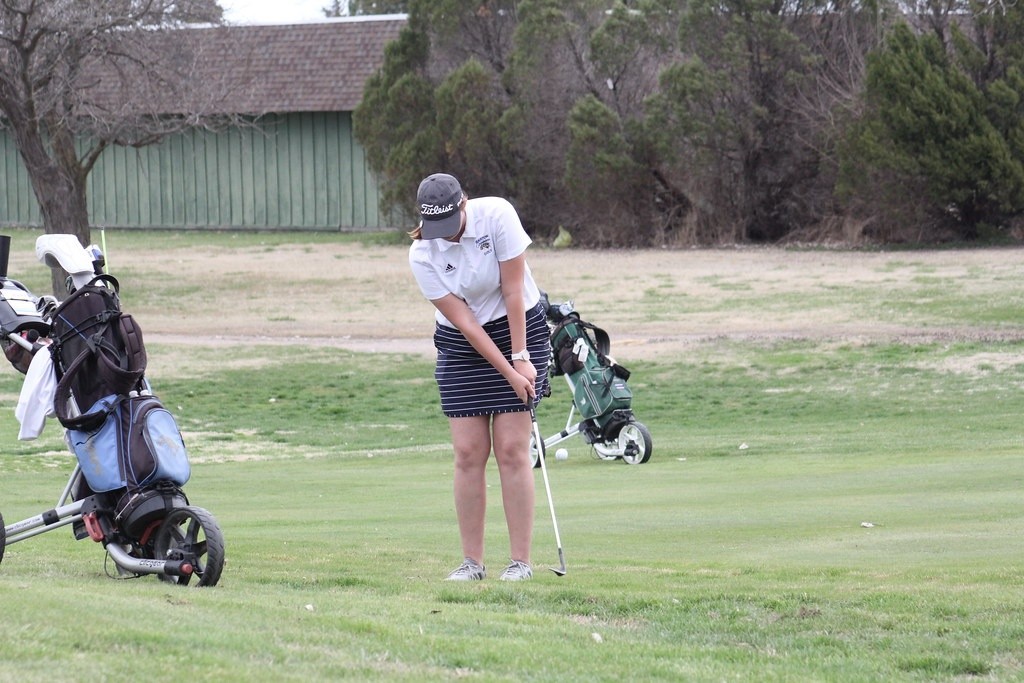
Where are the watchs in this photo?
[511,349,531,361]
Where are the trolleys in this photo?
[528,292,654,470]
[0,238,227,591]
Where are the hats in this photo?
[416,172,464,241]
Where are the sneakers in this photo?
[500,560,533,580]
[447,558,486,581]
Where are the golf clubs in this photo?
[525,389,566,577]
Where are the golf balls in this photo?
[556,449,568,460]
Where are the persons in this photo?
[408,173,552,586]
